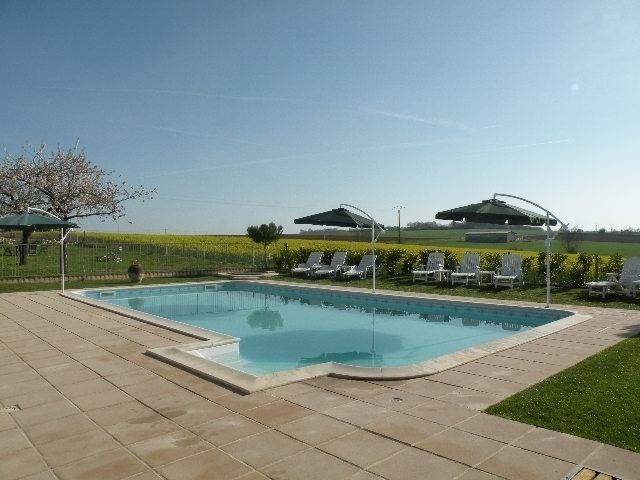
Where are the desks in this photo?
[585,281,615,299]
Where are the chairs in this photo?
[606,256,640,299]
[410,251,523,290]
[290,250,377,282]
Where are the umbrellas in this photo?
[435,193,568,308]
[0,207,81,293]
[294,204,386,292]
